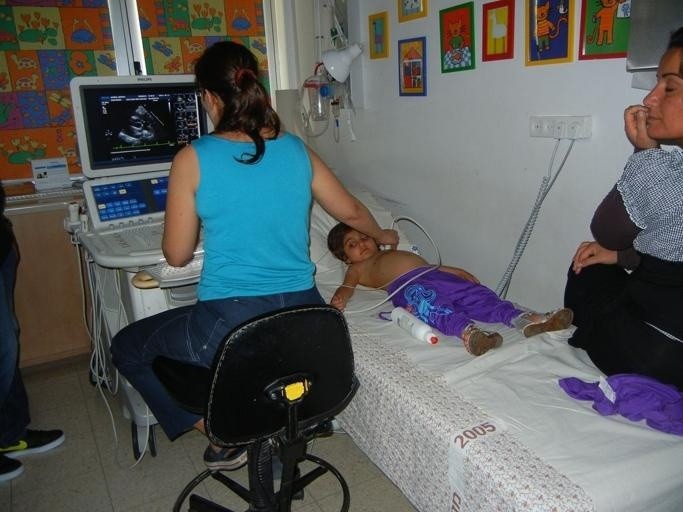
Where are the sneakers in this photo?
[0,429,65,459]
[0,453,24,482]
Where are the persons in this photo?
[110,40,401,471]
[568,26,683,388]
[0,174,66,485]
[326,214,576,356]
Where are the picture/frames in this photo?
[578,0,631,61]
[482,0,514,62]
[398,36,428,96]
[368,8,390,60]
[397,0,428,23]
[438,1,475,73]
[525,0,575,66]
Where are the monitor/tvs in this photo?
[69,74,216,181]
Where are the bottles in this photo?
[391,306,438,344]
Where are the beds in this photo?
[308,279,683,512]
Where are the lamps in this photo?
[313,0,364,84]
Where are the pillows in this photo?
[308,189,410,284]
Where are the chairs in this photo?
[173,305,360,511]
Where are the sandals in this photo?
[303,421,333,443]
[204,443,248,471]
[516,308,574,338]
[464,326,502,356]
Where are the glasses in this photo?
[194,84,218,104]
